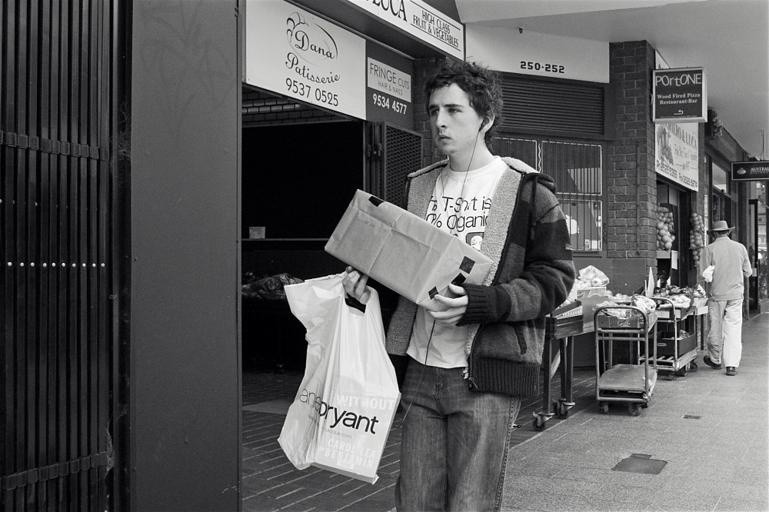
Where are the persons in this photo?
[699,220,752,376]
[340,63,575,511]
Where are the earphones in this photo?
[478,117,491,131]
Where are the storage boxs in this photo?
[325,189,494,315]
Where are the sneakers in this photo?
[703,355,722,368]
[727,367,736,374]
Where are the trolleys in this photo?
[593,297,659,416]
[654,288,702,377]
[531,298,584,431]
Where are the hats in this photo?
[707,221,736,233]
